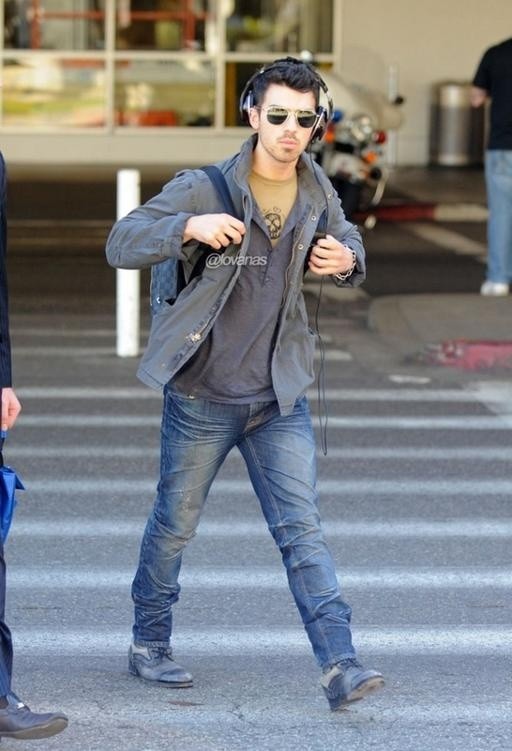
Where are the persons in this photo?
[104,54,388,715]
[467,34,512,298]
[0,156,70,740]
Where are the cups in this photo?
[257,105,320,128]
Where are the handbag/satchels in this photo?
[336,244,357,282]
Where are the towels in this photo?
[430,82,487,172]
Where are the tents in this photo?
[239,63,333,143]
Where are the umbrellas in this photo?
[320,657,386,712]
[128,641,194,688]
[0,692,68,740]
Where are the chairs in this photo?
[481,280,509,297]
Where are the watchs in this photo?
[301,50,402,229]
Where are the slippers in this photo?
[149,165,328,324]
